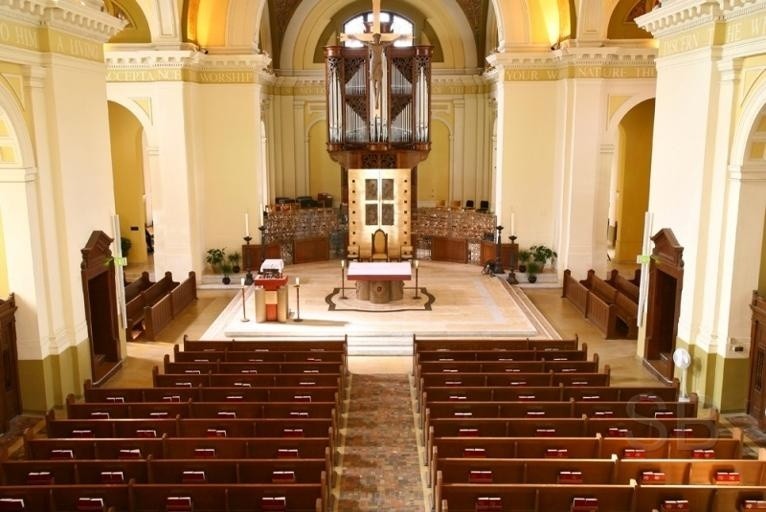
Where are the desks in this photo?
[346,262,412,304]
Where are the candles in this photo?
[416,260,419,268]
[260,203,263,226]
[246,214,249,236]
[511,213,515,236]
[341,260,345,267]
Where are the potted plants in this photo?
[518,245,557,283]
[206,247,242,285]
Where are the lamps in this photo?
[551,43,557,50]
[199,48,208,54]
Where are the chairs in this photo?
[347,245,360,268]
[370,229,390,262]
[399,245,414,265]
